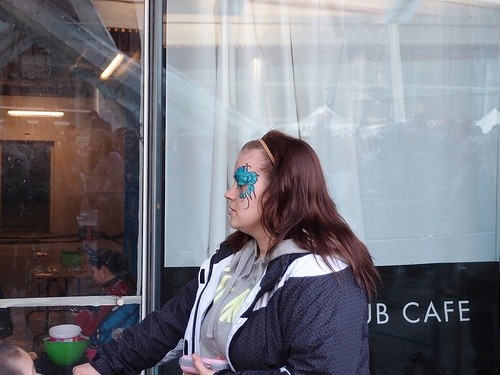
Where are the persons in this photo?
[72,130,382,375]
[71,248,132,338]
[0,343,43,375]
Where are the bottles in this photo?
[30,244,37,264]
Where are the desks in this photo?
[26,250,91,351]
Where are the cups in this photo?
[49,324,82,341]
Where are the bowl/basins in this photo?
[61,253,81,265]
[42,335,90,366]
